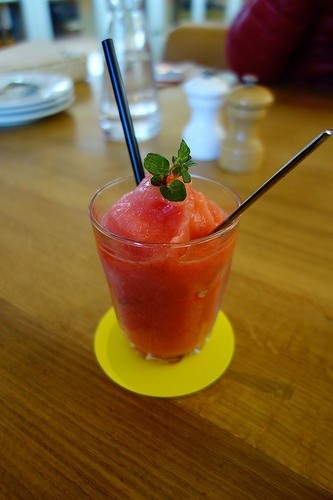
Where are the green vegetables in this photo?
[143,139,197,202]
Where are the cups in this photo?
[88,171,242,365]
[96,1,164,143]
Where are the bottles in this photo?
[218,75,275,174]
[181,65,240,162]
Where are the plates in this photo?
[0,70,75,127]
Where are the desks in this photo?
[0,79,333,500]
